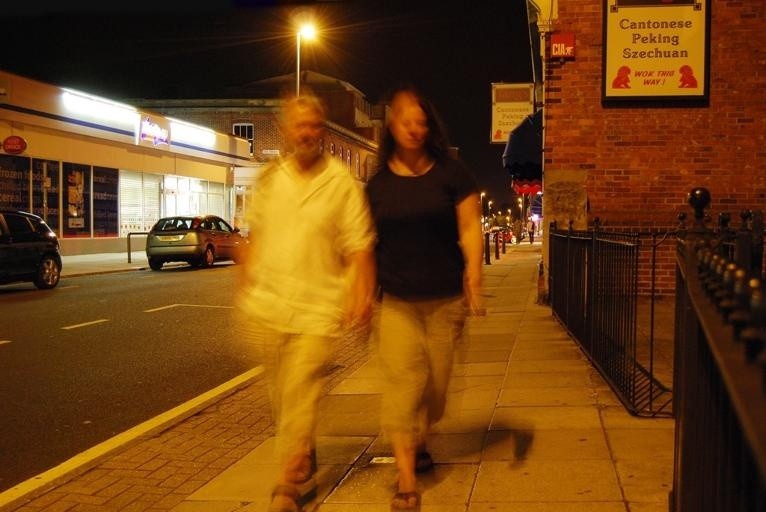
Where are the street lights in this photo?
[296,19,317,97]
[481,192,522,230]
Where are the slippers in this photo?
[390,451,434,511]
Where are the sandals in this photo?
[269,446,318,511]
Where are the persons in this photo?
[363,86,487,511]
[226,85,381,512]
[527,217,535,244]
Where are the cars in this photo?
[146,215,244,270]
[488,228,526,245]
[0,209,62,289]
